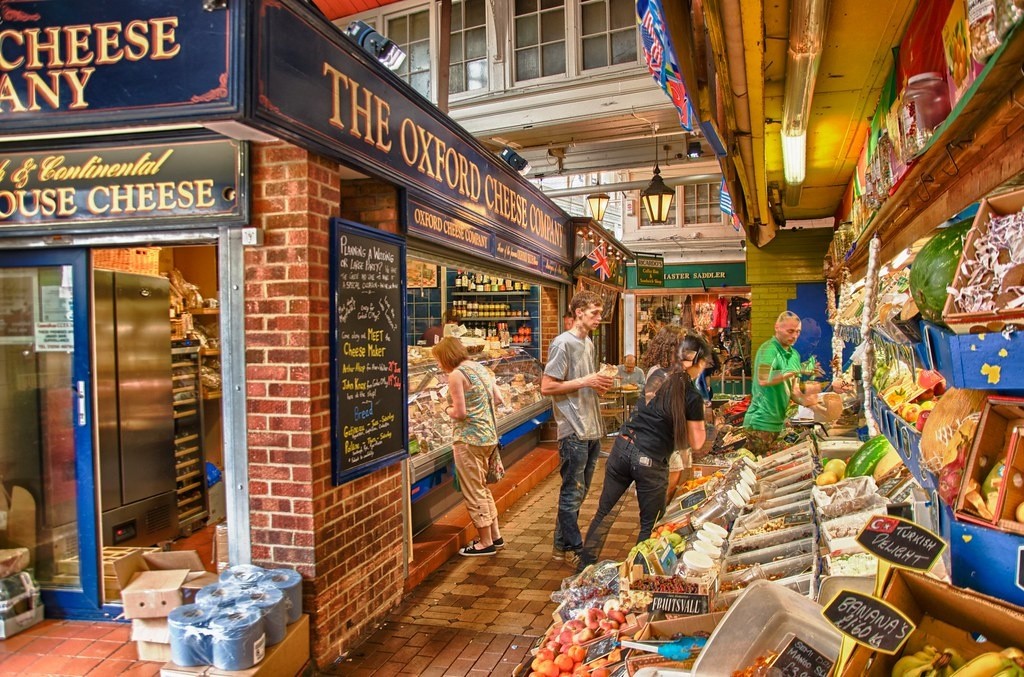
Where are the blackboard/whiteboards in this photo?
[581,632,619,666]
[891,481,919,503]
[783,430,800,445]
[606,573,619,598]
[631,549,651,574]
[874,477,907,497]
[608,663,627,677]
[769,635,835,677]
[679,490,708,510]
[783,513,812,525]
[329,214,410,487]
[854,513,948,574]
[820,588,916,656]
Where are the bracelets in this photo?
[445,406,453,415]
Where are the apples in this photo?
[815,458,847,486]
[1016,501,1024,522]
[888,382,946,432]
[529,599,642,677]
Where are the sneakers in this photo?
[552,546,597,561]
[469,538,505,547]
[458,541,496,556]
[565,550,592,571]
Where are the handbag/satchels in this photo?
[486,446,504,484]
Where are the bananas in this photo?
[948,647,1024,677]
[891,644,966,677]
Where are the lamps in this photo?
[345,20,406,72]
[640,137,675,225]
[686,142,704,159]
[587,181,610,222]
[500,147,532,177]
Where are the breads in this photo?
[408,337,536,450]
[170,268,222,392]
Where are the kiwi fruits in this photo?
[970,322,1024,334]
[980,247,1024,309]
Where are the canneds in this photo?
[513,326,531,343]
[524,310,529,317]
[832,72,952,266]
[452,300,522,317]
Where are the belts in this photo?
[621,433,636,444]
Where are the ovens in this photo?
[91,268,181,549]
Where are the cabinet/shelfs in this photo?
[186,308,221,399]
[451,291,531,346]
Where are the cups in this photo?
[613,379,621,389]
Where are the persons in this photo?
[743,311,821,453]
[616,354,645,390]
[792,318,822,382]
[541,291,614,572]
[432,337,505,556]
[577,334,706,574]
[641,324,714,507]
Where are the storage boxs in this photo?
[0,484,310,677]
[513,185,1024,677]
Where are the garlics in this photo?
[860,238,881,439]
[831,282,852,380]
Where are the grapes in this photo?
[629,573,699,593]
[562,561,619,620]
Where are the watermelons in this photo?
[844,434,891,478]
[909,216,974,326]
[981,458,1006,501]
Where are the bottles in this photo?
[450,269,532,346]
[651,457,757,578]
